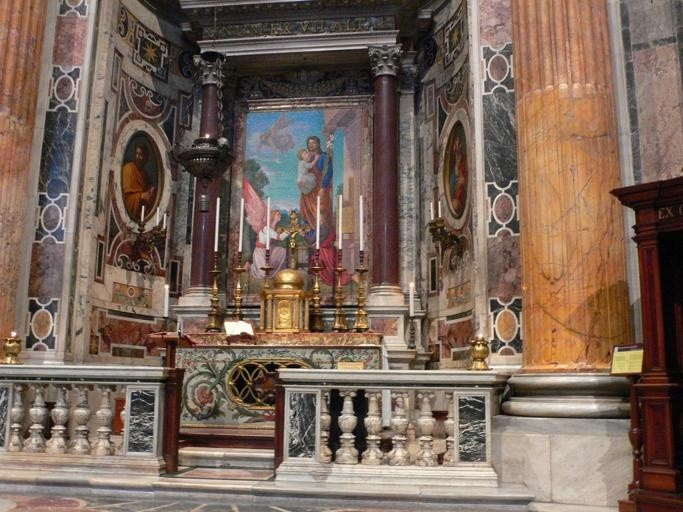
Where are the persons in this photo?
[243,137,351,286]
[122,144,156,219]
[451,136,467,213]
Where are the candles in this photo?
[214,198,220,252]
[163,214,166,229]
[141,205,145,222]
[157,207,159,225]
[239,198,245,252]
[164,285,169,317]
[438,200,441,217]
[266,197,270,250]
[339,195,342,249]
[410,282,414,316]
[359,195,363,251]
[430,202,433,220]
[316,196,320,249]
